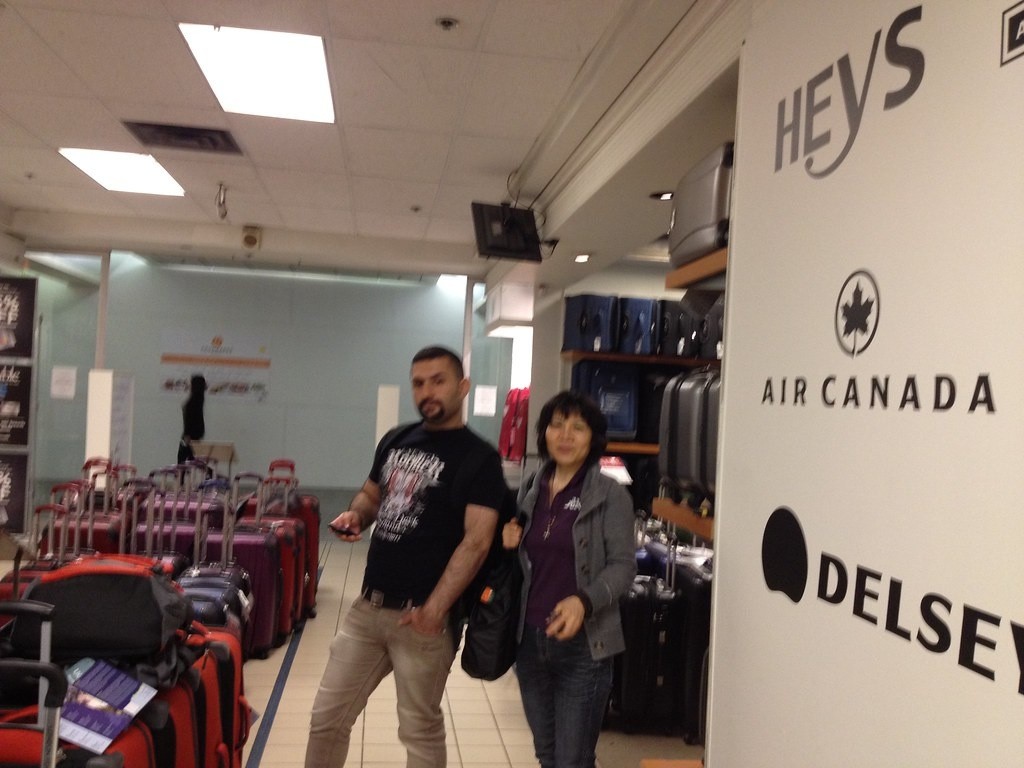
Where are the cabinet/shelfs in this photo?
[1,269,39,567]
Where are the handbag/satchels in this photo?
[462,560,523,680]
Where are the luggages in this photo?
[563,141,733,749]
[0,459,321,768]
[496,386,530,463]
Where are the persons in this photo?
[304,347,508,767]
[176,374,208,483]
[501,389,637,768]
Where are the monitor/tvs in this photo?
[470,201,542,262]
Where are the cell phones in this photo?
[328,523,356,535]
